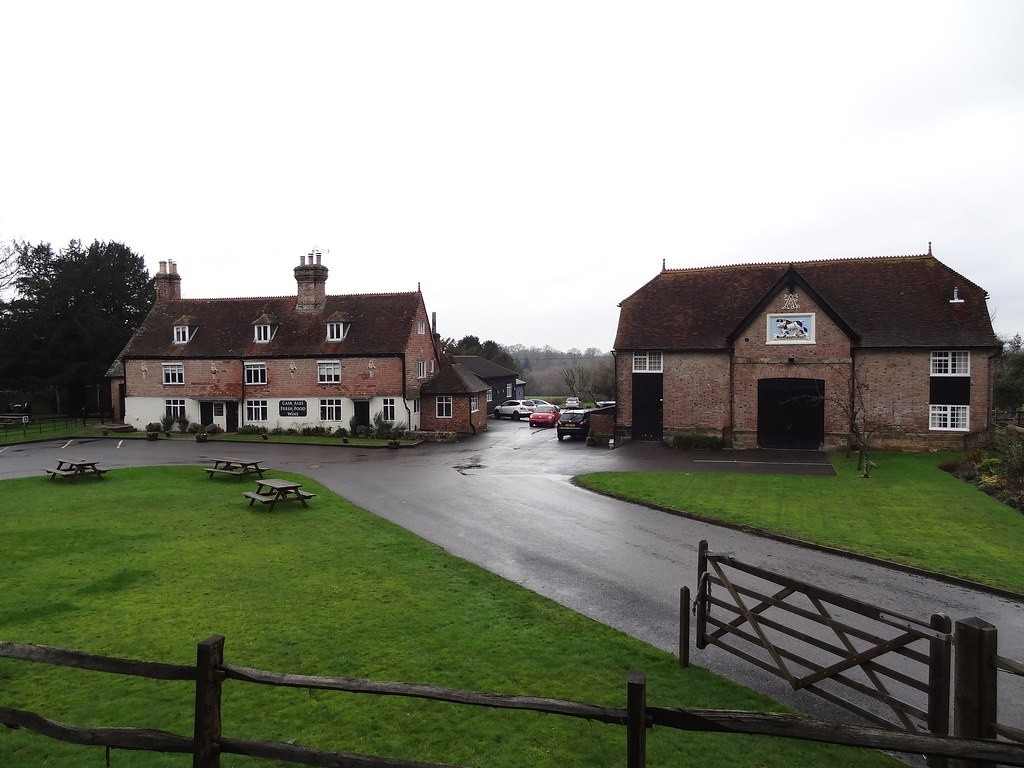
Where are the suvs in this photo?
[596,400,617,409]
[494,400,536,421]
[556,408,598,441]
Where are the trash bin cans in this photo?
[964,431,979,452]
[722,426,733,447]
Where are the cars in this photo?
[529,405,562,428]
[565,396,584,409]
[528,399,561,412]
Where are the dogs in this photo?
[388,439,400,449]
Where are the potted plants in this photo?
[388,430,401,450]
[147,423,158,441]
[195,424,208,442]
[586,427,595,446]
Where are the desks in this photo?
[50,458,104,484]
[249,479,309,513]
[208,458,264,481]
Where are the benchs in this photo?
[204,468,245,476]
[287,490,317,499]
[42,467,74,479]
[231,465,271,472]
[242,491,273,505]
[85,466,111,474]
